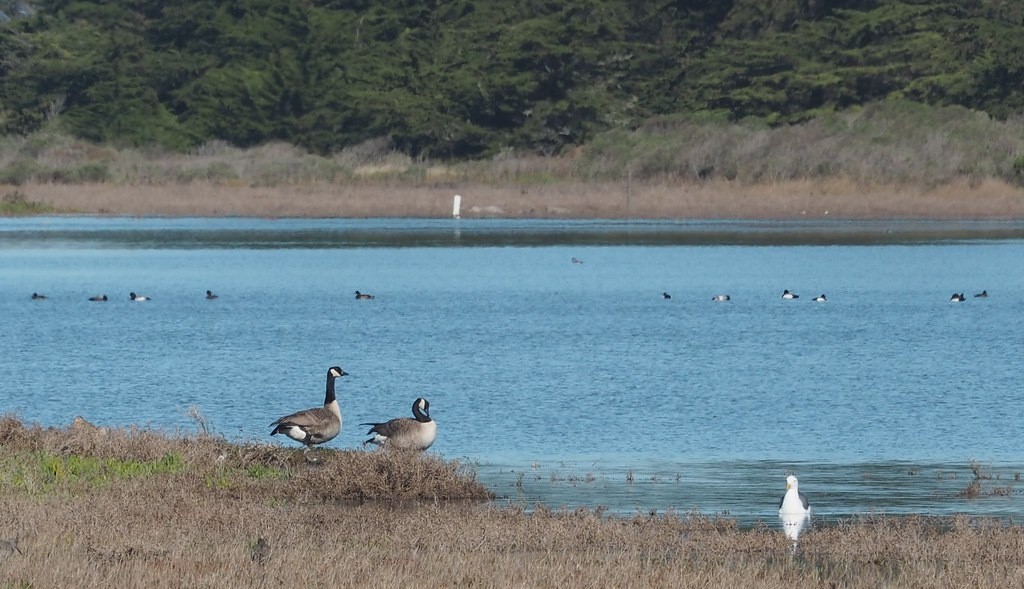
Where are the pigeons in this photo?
[780,474,813,567]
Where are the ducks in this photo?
[28,248,995,318]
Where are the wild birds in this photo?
[268,365,349,455]
[358,398,436,451]
